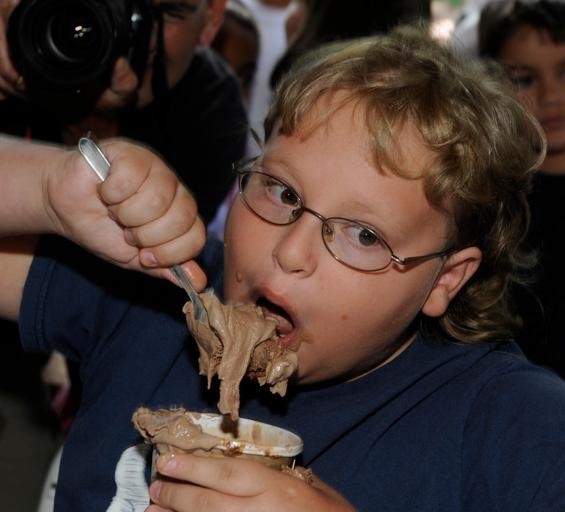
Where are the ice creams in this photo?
[130,291,313,484]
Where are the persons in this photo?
[1,17,565,511]
[256,1,460,116]
[0,0,250,242]
[469,0,565,380]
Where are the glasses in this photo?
[230,155,452,274]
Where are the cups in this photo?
[147,411,306,512]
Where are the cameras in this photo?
[6,0,155,117]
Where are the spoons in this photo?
[74,134,298,381]
